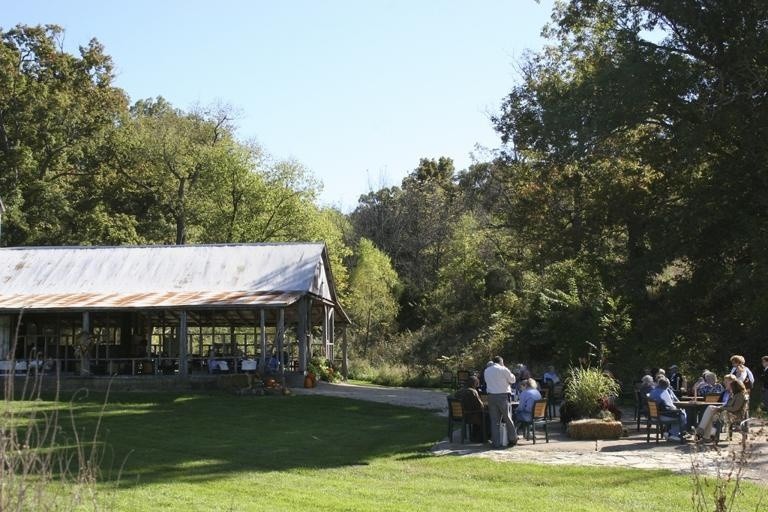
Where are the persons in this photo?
[453,356,560,450]
[137,335,147,358]
[638,355,755,444]
[761,356,768,408]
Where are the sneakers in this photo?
[510,439,518,445]
[488,440,497,447]
[664,430,713,445]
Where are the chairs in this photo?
[438,370,563,444]
[633,376,753,440]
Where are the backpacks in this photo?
[670,373,682,389]
[745,368,754,389]
